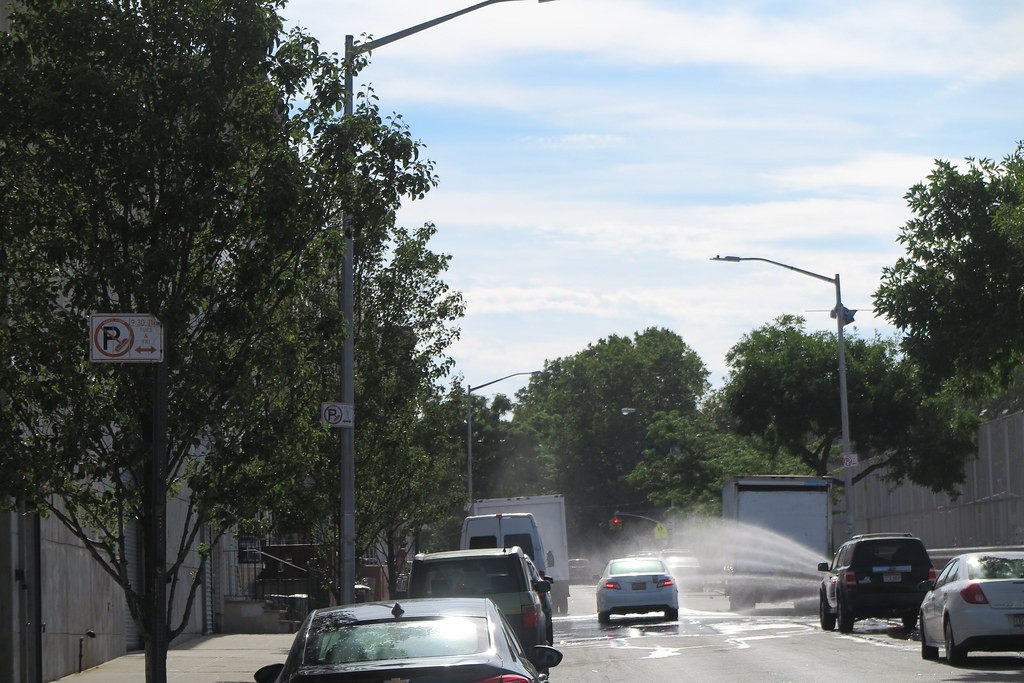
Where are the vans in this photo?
[393,547,552,683]
[460,511,555,600]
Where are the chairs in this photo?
[891,547,911,563]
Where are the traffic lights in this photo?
[608,519,615,531]
[613,519,618,525]
[617,520,622,530]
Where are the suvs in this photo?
[817,532,937,633]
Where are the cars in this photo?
[252,597,563,683]
[592,557,679,624]
[625,547,704,593]
[523,552,555,647]
[916,550,1024,666]
[568,558,592,585]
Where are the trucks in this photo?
[473,493,571,616]
[721,473,834,615]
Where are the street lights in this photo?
[467,370,541,502]
[708,255,854,541]
[337,0,555,606]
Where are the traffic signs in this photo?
[90,312,163,363]
[321,402,354,429]
